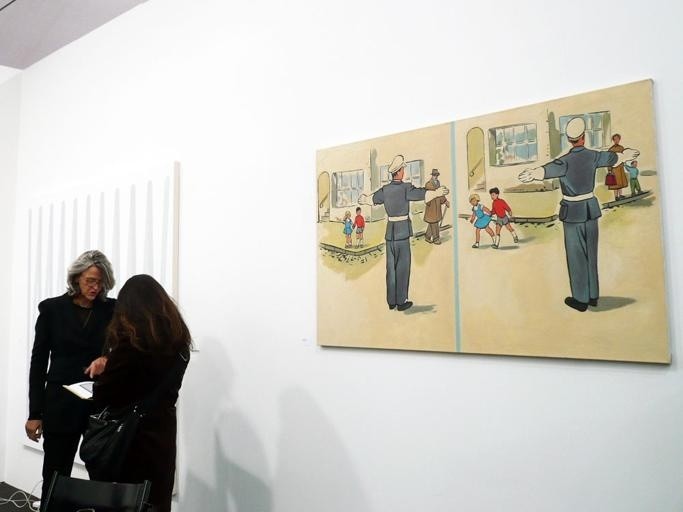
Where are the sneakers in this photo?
[491,244,498,249]
[472,244,479,248]
[514,236,518,243]
[616,195,625,200]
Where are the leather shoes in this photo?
[425,237,441,244]
[565,297,597,311]
[389,301,412,311]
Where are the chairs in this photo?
[40,470,150,511]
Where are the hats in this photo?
[389,155,406,174]
[431,169,440,175]
[565,117,586,141]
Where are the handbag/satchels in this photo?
[80,406,141,470]
[606,174,617,185]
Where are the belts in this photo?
[563,192,594,202]
[388,215,408,221]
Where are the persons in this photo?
[80,274,194,510]
[489,188,518,248]
[343,210,354,248]
[518,117,641,312]
[623,161,641,196]
[608,134,628,201]
[424,169,450,244]
[25,250,117,511]
[468,194,496,248]
[358,155,449,310]
[352,207,365,248]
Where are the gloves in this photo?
[425,186,448,203]
[612,149,639,167]
[358,194,376,206]
[519,166,545,182]
